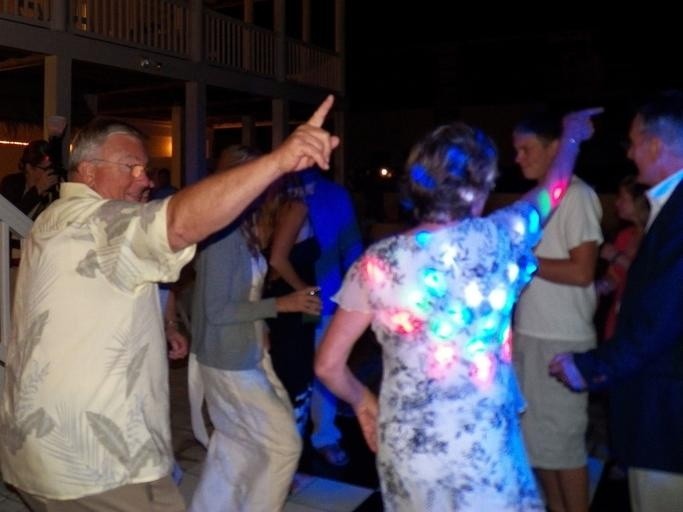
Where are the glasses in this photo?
[90,158,146,178]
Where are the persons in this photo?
[2,140,65,309]
[151,144,365,512]
[2,95,339,512]
[512,100,683,511]
[314,108,602,512]
